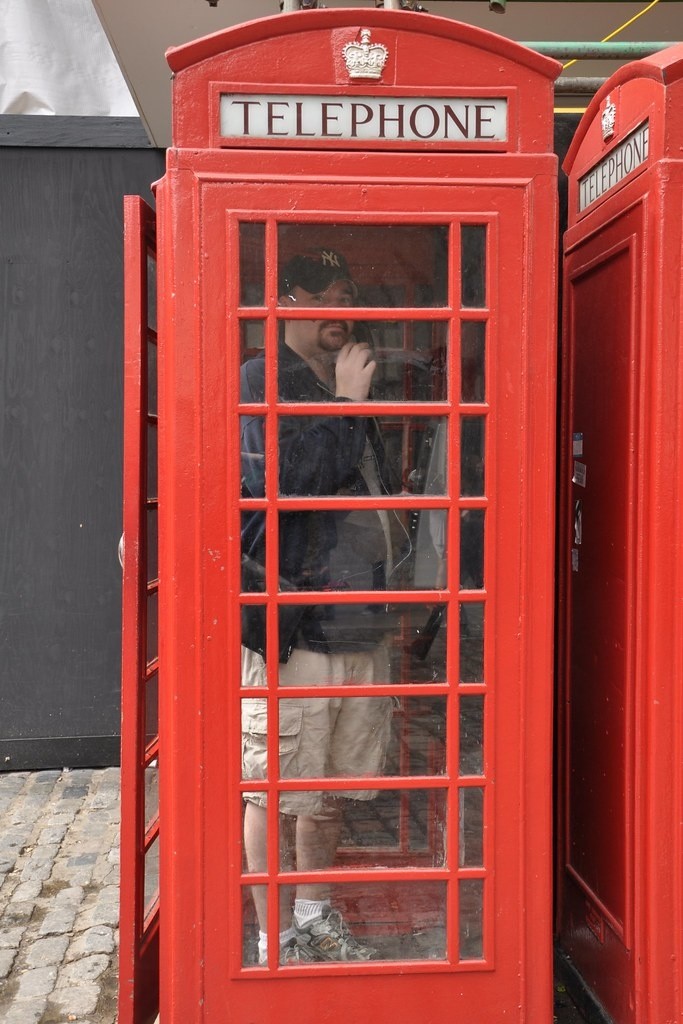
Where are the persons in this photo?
[237,249,416,968]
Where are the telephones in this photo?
[351,294,375,369]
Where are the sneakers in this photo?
[292,904,383,961]
[257,937,333,964]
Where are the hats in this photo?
[278,247,358,300]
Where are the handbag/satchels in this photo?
[243,557,306,664]
[301,612,388,654]
